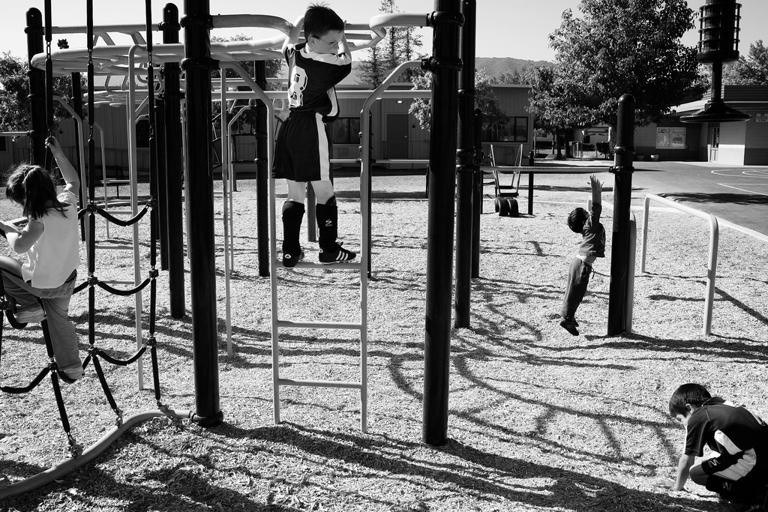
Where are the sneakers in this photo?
[559,316,579,336]
[561,314,579,327]
[282,246,305,267]
[47,364,86,381]
[318,240,357,263]
[5,307,47,325]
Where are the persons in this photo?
[0,134,86,380]
[272,4,357,267]
[667,383,768,512]
[559,175,607,337]
